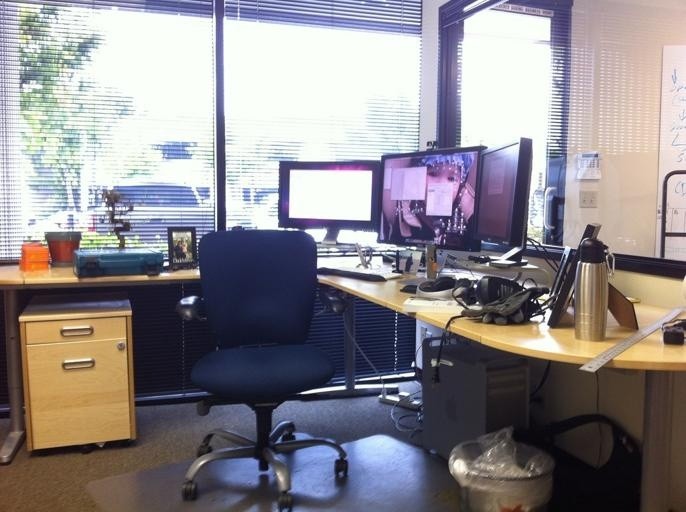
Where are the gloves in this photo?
[461,286,550,326]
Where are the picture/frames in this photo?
[165,225,197,270]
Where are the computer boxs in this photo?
[422,336,530,461]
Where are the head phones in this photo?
[416,276,471,299]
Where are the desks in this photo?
[314,240,686,510]
[0,260,203,464]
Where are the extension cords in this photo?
[378,394,422,410]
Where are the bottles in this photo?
[574,237,609,342]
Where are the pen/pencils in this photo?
[396,250,399,271]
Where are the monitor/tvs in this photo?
[377,146,488,252]
[317,266,403,282]
[472,137,533,268]
[278,161,381,249]
[542,156,567,246]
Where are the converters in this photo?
[398,392,410,401]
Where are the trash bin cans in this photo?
[451,438,556,512]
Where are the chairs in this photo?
[177,226,349,511]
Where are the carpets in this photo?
[82,430,469,512]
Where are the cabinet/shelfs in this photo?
[18,287,136,454]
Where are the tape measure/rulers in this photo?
[578,305,685,374]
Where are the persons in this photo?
[181,240,187,260]
[174,241,181,262]
[382,153,475,246]
